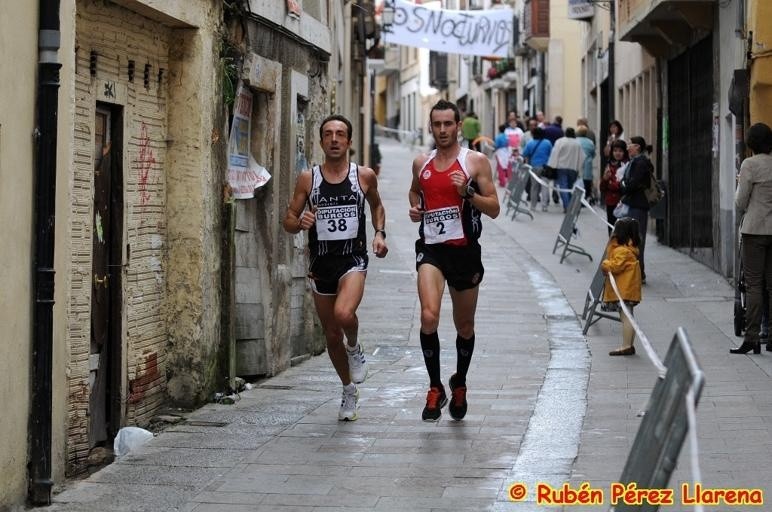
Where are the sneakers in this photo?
[337,387,360,423]
[449,374,468,421]
[421,386,449,423]
[344,340,370,385]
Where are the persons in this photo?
[461,111,595,213]
[730,123,772,354]
[602,217,642,355]
[620,136,654,284]
[284,116,388,421]
[603,140,630,238]
[604,121,631,156]
[409,100,499,422]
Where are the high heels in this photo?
[729,341,761,355]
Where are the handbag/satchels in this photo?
[643,174,665,204]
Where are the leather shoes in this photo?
[608,344,636,356]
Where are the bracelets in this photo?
[375,229,386,238]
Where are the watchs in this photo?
[462,186,475,200]
